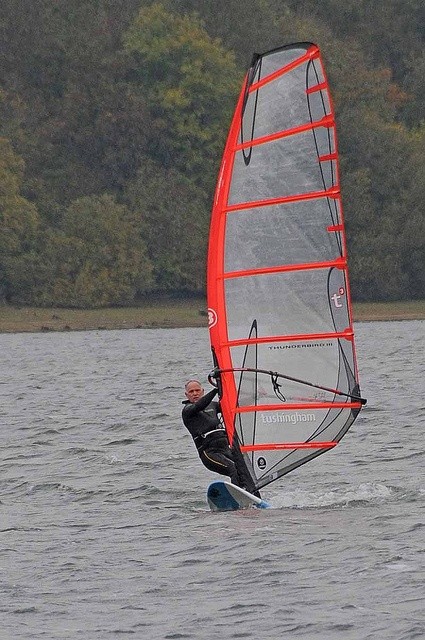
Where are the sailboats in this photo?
[206,40,367,512]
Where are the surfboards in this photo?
[207,482,271,512]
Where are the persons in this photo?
[182,379,264,500]
